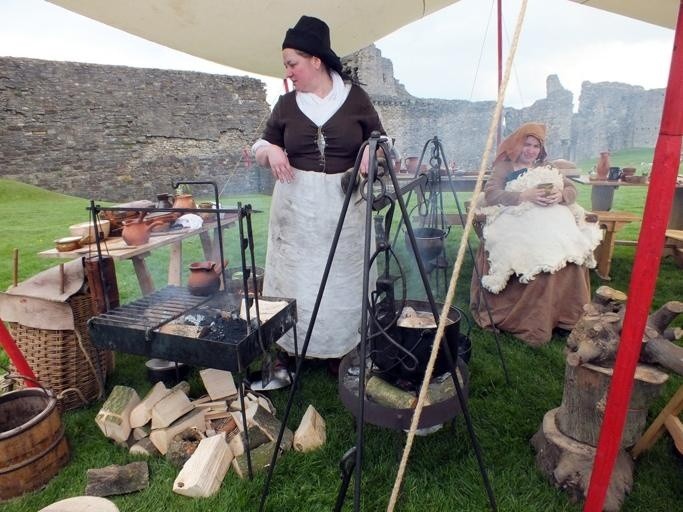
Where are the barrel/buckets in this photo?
[0,364,70,501]
[225,266,266,295]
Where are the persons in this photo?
[469,121,608,347]
[250,13,389,379]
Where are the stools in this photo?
[661,226,683,260]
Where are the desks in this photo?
[566,166,683,233]
[35,202,247,301]
[393,163,511,228]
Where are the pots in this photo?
[447,305,475,365]
[371,298,461,382]
[402,225,453,261]
[146,359,186,387]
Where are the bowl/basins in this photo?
[623,168,636,177]
[70,219,110,243]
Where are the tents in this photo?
[0,0,683,507]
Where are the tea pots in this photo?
[198,202,218,223]
[405,157,419,174]
[156,192,175,210]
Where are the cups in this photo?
[538,184,554,206]
[609,166,622,180]
[421,164,428,173]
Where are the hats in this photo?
[282,15,343,72]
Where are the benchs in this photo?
[466,196,643,283]
